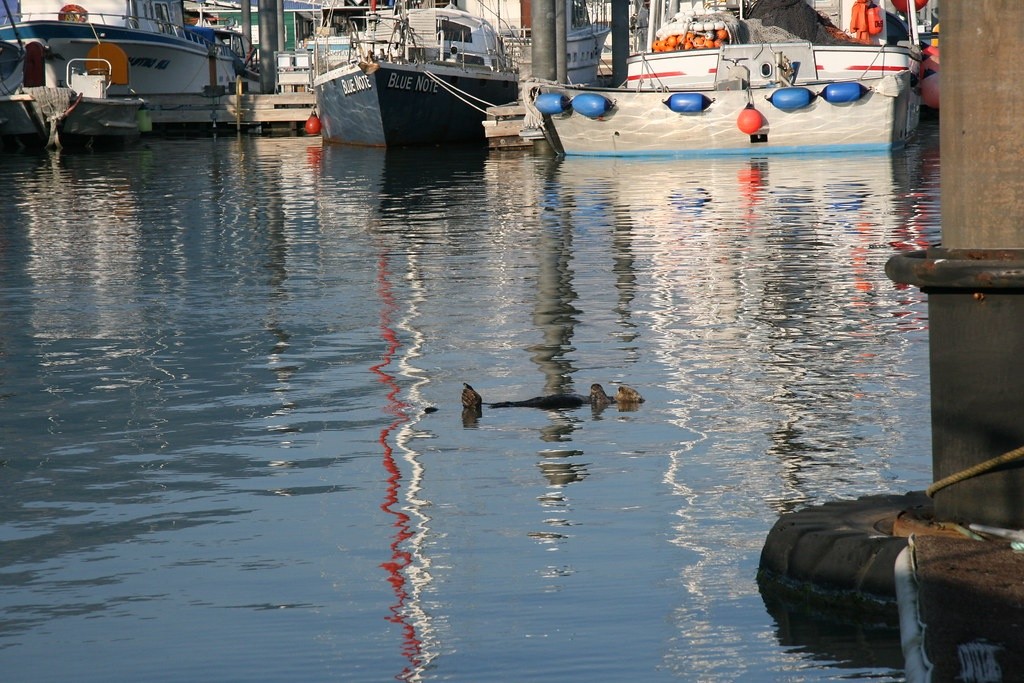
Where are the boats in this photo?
[1,0,941,156]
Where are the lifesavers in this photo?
[891,0,929,13]
[58,5,89,23]
